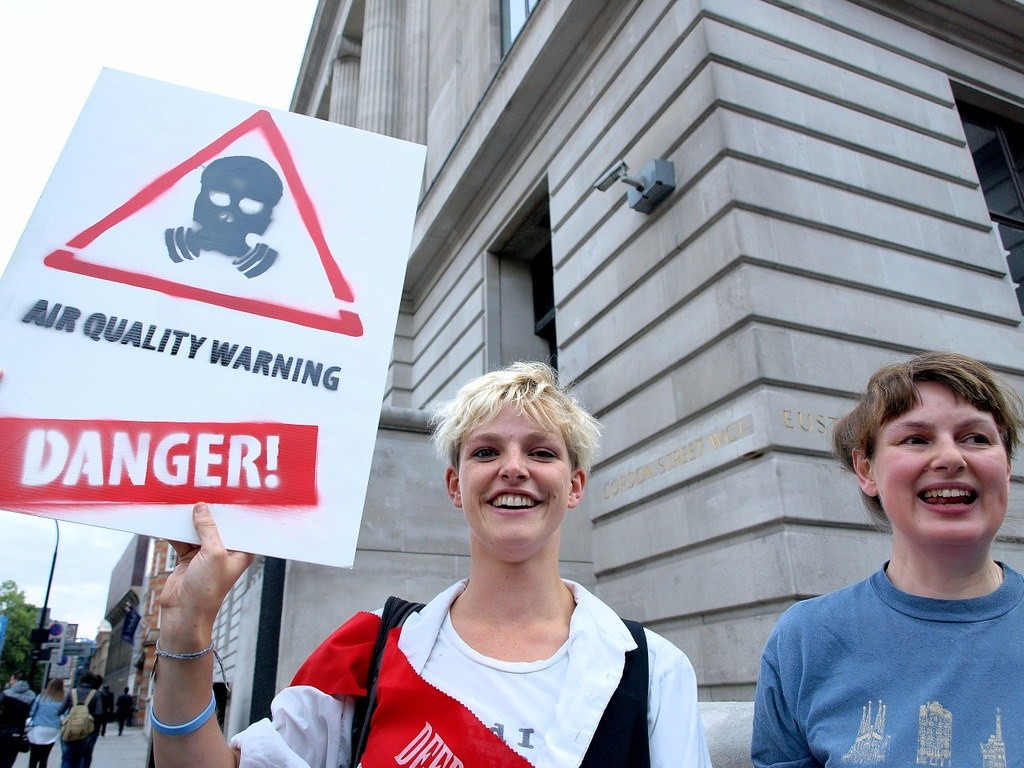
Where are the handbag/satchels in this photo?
[18,733,31,753]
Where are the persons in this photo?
[90,678,135,738]
[1,669,104,768]
[149,359,713,768]
[749,349,1024,767]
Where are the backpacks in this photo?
[60,689,96,741]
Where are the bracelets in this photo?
[148,688,221,735]
[153,634,236,698]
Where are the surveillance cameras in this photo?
[593,159,629,191]
